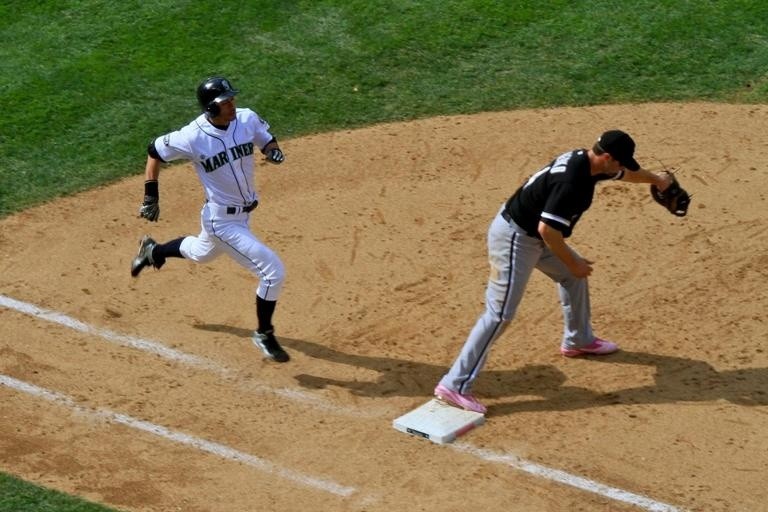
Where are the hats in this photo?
[598,129,640,171]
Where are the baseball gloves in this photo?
[650,170,690,216]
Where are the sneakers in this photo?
[434,383,488,416]
[129,236,161,277]
[560,334,617,358]
[251,324,289,363]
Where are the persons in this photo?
[433,130,675,412]
[129,75,291,363]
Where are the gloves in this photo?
[139,180,160,222]
[264,147,285,165]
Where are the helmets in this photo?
[195,76,241,120]
